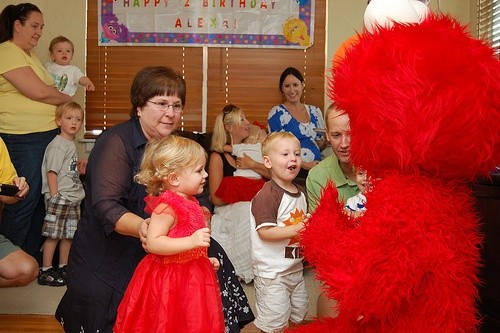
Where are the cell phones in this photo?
[0,184,19,196]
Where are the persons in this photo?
[46,35,95,97]
[1,3,72,268]
[0,66,376,333]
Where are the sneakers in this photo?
[38,267,66,286]
[56,266,68,279]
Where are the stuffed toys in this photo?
[281,0,500,333]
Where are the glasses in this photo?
[222,103,237,121]
[146,98,184,113]
